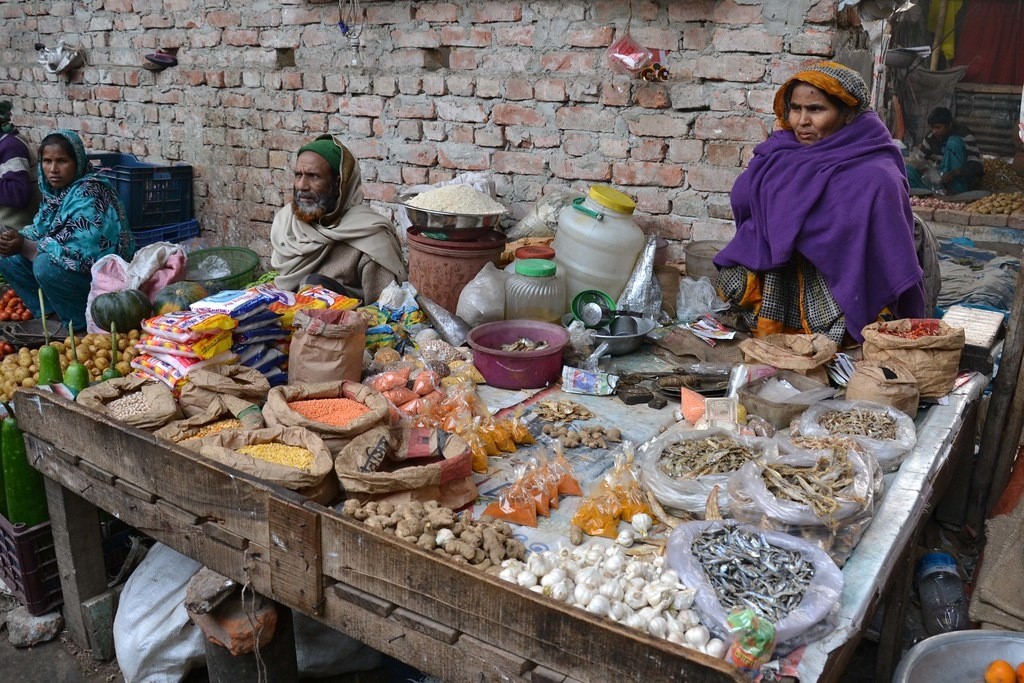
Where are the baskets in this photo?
[184,246,259,295]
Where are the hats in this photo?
[298,140,342,174]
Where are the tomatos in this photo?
[0,290,32,360]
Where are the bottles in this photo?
[917,545,967,635]
[551,186,645,313]
[504,246,555,274]
[505,258,565,325]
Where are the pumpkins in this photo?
[153,281,206,316]
[91,288,152,333]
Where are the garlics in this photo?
[500,514,726,660]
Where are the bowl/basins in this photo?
[885,50,917,67]
[562,313,655,356]
[393,192,507,242]
[892,629,1024,683]
[468,320,569,390]
[569,289,617,328]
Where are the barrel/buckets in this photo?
[654,266,679,317]
[406,237,507,315]
[683,239,731,296]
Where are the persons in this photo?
[906,107,983,198]
[0,129,137,341]
[270,134,408,306]
[712,62,926,357]
[0,100,47,232]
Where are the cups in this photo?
[654,237,669,266]
[609,317,638,335]
[582,303,611,326]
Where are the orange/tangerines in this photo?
[1016,662,1024,683]
[985,660,1016,683]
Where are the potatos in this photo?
[0,329,140,400]
